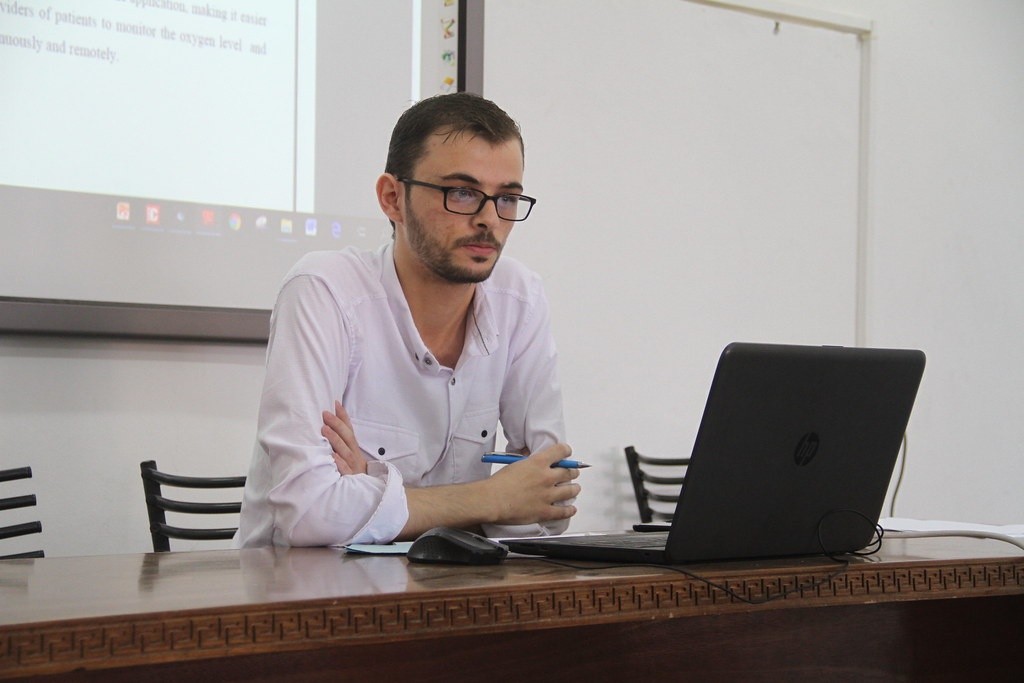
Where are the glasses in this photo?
[396,177,536,221]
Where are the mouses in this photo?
[406,526,509,564]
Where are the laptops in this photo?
[499,343,927,565]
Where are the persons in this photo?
[230,93,581,550]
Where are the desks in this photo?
[0,517,1024,683]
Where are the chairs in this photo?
[139,459,247,552]
[623,446,689,524]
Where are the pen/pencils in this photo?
[481,451,592,469]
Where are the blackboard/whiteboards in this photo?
[484,0,874,542]
